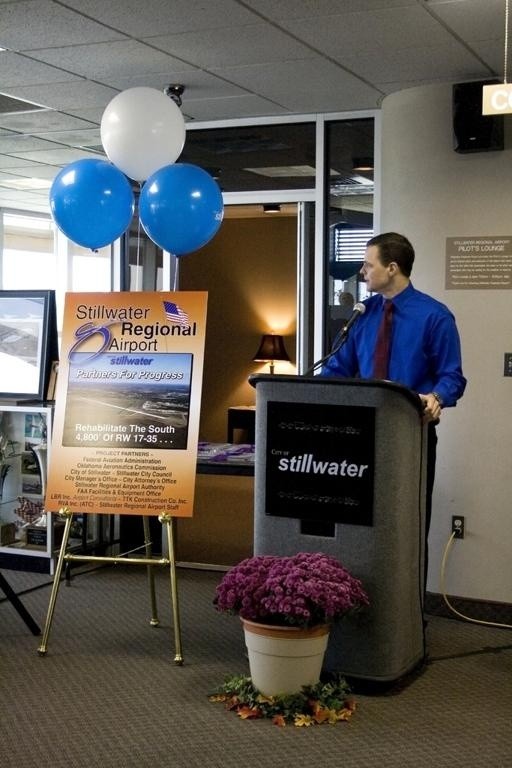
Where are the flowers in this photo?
[211,549,373,631]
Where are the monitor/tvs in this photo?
[0,289,52,403]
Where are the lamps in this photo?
[254,334,292,374]
[261,202,282,215]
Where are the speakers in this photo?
[451,79,504,154]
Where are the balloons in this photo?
[137,163,225,258]
[49,157,135,249]
[98,84,187,181]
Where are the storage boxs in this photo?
[23,517,70,551]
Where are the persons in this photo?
[313,231,468,639]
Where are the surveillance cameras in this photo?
[164,85,186,107]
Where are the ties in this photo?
[369,300,395,383]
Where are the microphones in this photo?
[340,302,366,336]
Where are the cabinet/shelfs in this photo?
[0,400,101,573]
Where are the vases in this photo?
[236,608,334,700]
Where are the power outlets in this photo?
[451,513,465,540]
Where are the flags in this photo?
[162,299,188,326]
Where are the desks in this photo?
[226,404,256,442]
